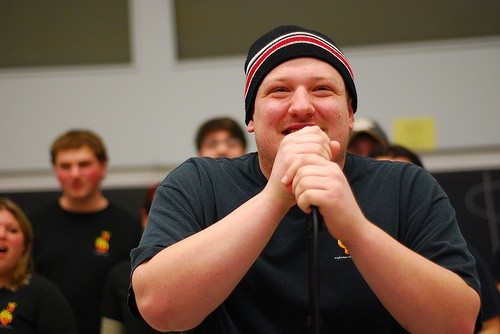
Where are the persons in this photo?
[0,197,73,334]
[28,129,149,334]
[347,117,500,334]
[127,25,485,334]
[194,114,248,157]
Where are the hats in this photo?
[244,25,358,124]
